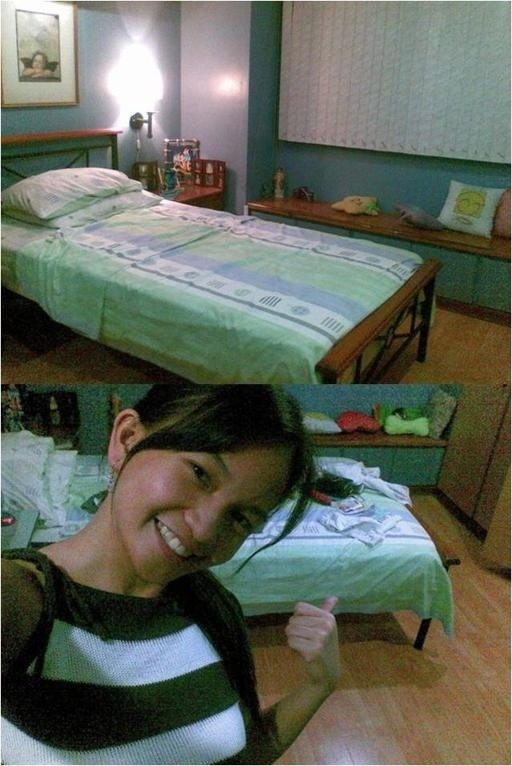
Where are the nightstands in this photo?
[146,185,223,210]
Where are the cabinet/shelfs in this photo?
[312,448,445,487]
[436,384,510,534]
[245,211,511,319]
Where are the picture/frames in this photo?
[1,2,80,110]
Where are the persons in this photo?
[330,191,381,217]
[21,48,54,83]
[1,374,342,765]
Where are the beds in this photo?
[32,453,460,652]
[2,128,441,384]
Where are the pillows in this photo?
[2,166,163,230]
[394,177,509,239]
[1,429,79,530]
